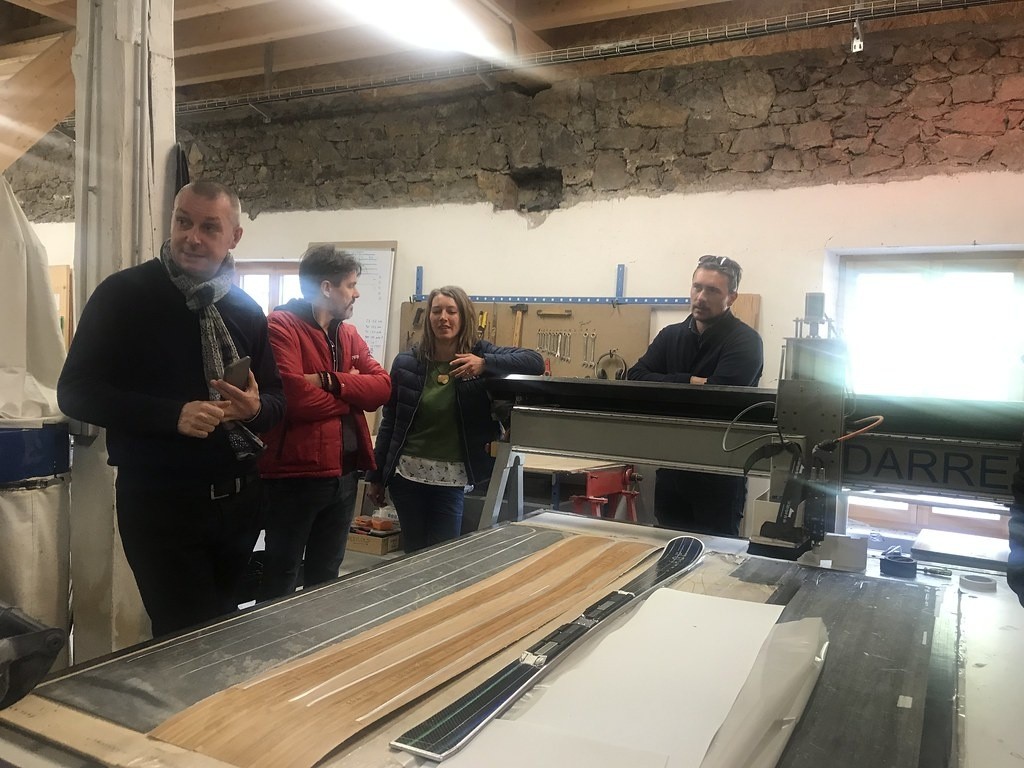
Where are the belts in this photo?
[210,478,241,500]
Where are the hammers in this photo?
[510,304,528,348]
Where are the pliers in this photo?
[477,310,488,339]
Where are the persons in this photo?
[627,255,764,536]
[253,243,392,604]
[57,180,287,639]
[365,285,546,554]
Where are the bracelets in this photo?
[319,368,330,392]
[242,401,262,423]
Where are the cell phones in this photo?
[221,355,251,402]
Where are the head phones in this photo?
[595,352,627,381]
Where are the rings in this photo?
[466,371,470,375]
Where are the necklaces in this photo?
[431,364,450,385]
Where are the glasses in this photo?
[698,255,737,292]
